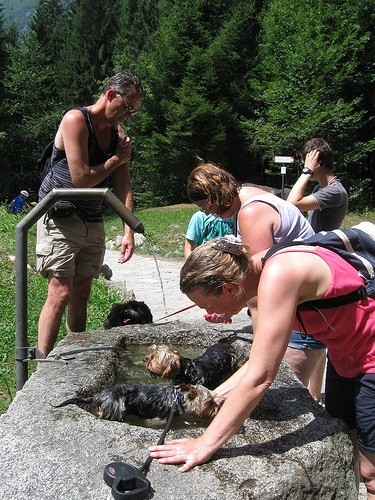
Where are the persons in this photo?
[36,72,146,357]
[184,210,234,324]
[286,138,348,402]
[187,163,328,387]
[9,190,29,215]
[149,235,375,500]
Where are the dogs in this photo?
[103,298,155,331]
[48,380,222,428]
[142,330,241,390]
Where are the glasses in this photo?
[200,201,209,212]
[115,89,139,116]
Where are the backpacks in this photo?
[260,221,375,311]
[37,106,94,184]
[7,199,15,214]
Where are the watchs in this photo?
[302,167,314,177]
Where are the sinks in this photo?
[75,329,243,435]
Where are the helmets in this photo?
[30,202,38,207]
[20,190,29,197]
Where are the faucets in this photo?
[15,187,145,361]
